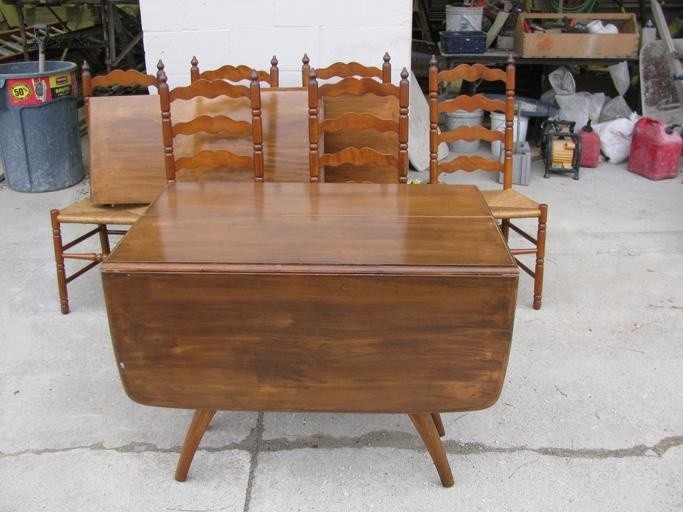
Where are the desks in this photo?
[99,180,522,488]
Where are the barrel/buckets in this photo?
[488,109,528,160]
[445,3,485,33]
[575,122,600,168]
[443,109,483,153]
[625,115,682,180]
[1,60,86,192]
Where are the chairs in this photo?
[159,52,410,205]
[428,51,549,311]
[50,59,166,314]
